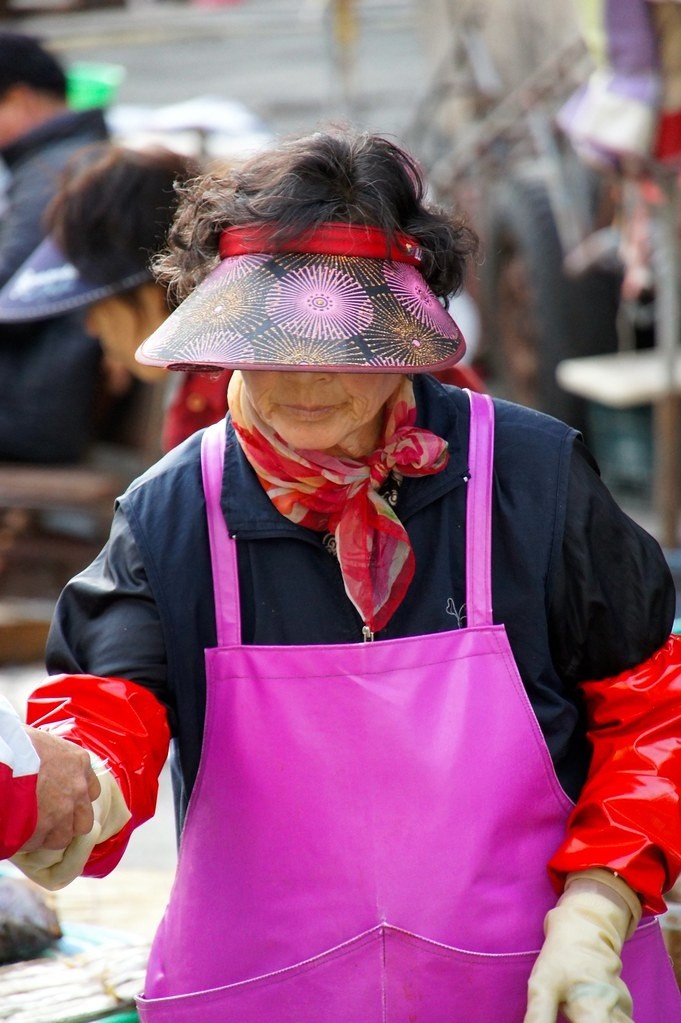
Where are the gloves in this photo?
[522,868,642,1023]
[7,747,132,891]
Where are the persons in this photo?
[9,133,681,1023]
[0,694,102,859]
[0,33,234,587]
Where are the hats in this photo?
[0,236,152,322]
[133,221,467,374]
